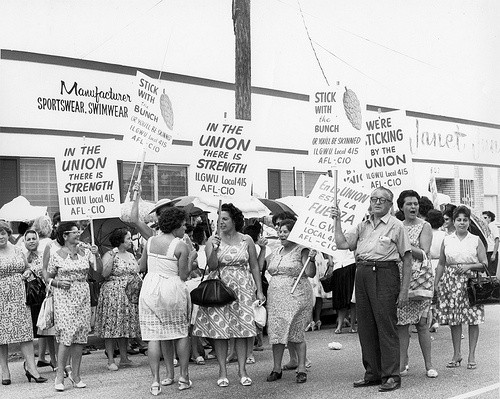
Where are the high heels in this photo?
[2,358,86,391]
[305,321,321,332]
[429,323,439,333]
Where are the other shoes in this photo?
[353,358,476,392]
[335,325,358,334]
[149,353,312,397]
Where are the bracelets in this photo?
[57,280,59,288]
[310,260,315,263]
[96,257,100,259]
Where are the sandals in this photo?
[119,360,141,367]
[106,363,118,371]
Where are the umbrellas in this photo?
[194,193,309,219]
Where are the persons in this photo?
[434,203,488,369]
[193,202,265,387]
[306,247,358,335]
[394,189,448,379]
[132,179,199,395]
[94,227,143,370]
[335,186,412,392]
[15,215,52,368]
[261,212,317,384]
[480,210,499,275]
[41,211,103,392]
[0,221,48,385]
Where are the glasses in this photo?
[64,230,81,235]
[273,222,280,227]
[370,195,392,204]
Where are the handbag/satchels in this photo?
[37,277,61,333]
[87,279,99,307]
[189,280,238,306]
[126,279,141,304]
[25,268,46,306]
[408,249,433,301]
[467,261,499,305]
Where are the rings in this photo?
[64,287,66,289]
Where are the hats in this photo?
[183,203,211,218]
[148,198,182,215]
